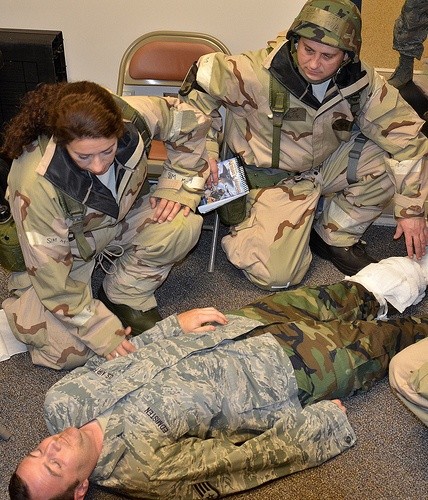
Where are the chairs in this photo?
[116,29,232,273]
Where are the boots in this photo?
[386,53,414,87]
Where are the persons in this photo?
[386,0,428,87]
[8,252,428,500]
[0,81,210,371]
[178,0,428,289]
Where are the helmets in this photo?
[286,0,362,64]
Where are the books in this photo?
[197,155,250,214]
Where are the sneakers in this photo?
[309,226,377,273]
[98,284,163,334]
